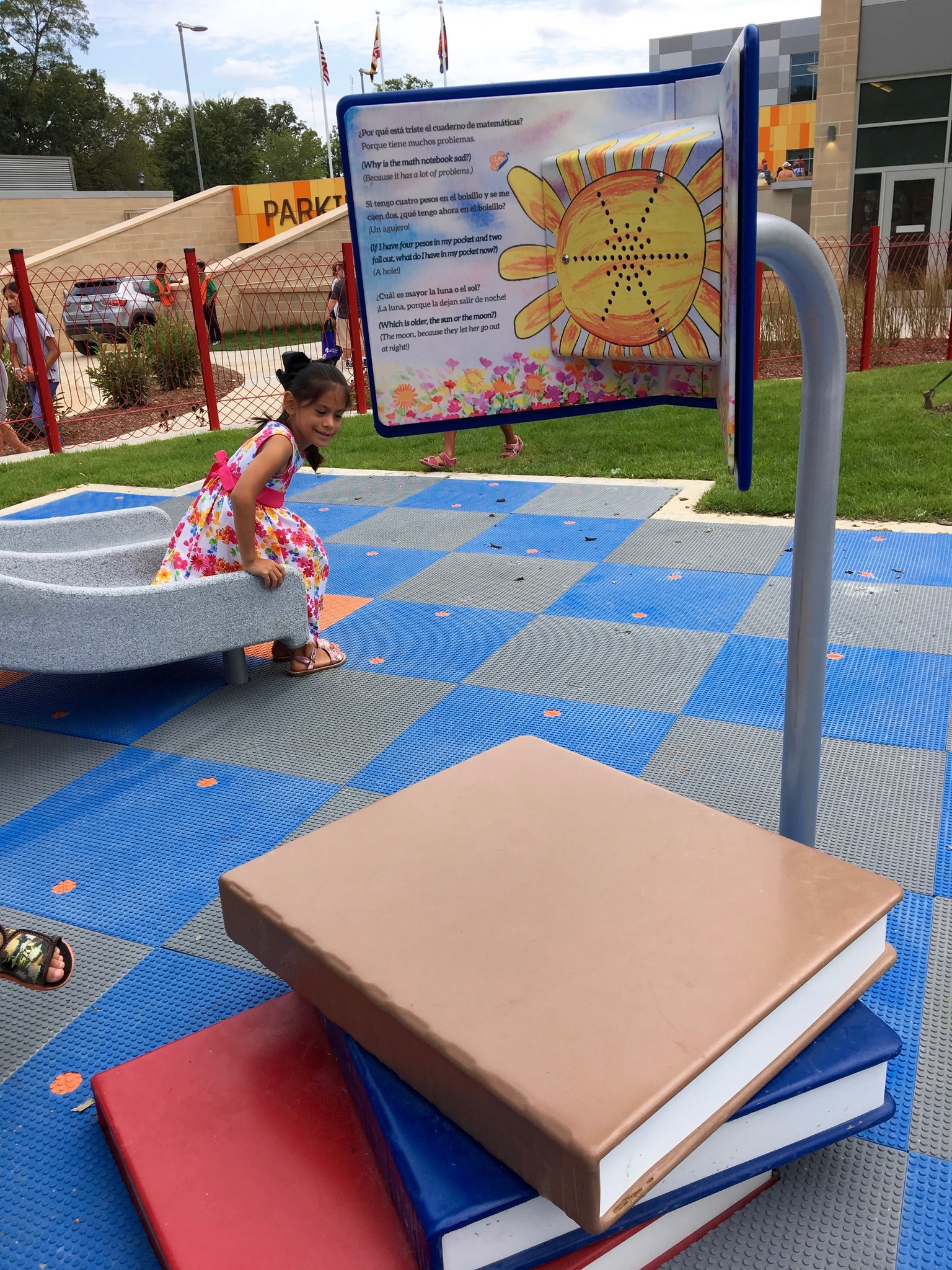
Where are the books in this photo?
[89,989,779,1270]
[313,996,904,1270]
[217,729,900,1233]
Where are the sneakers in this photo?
[345,357,354,368]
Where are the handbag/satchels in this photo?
[329,291,336,320]
[321,318,343,360]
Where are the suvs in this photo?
[62,275,158,356]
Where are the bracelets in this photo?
[15,367,23,374]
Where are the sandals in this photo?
[418,452,457,472]
[271,638,339,661]
[0,924,74,991]
[287,645,346,677]
[500,435,524,459]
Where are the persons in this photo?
[324,261,367,368]
[0,924,73,991]
[417,424,524,470]
[152,362,352,677]
[197,261,221,345]
[1,282,65,448]
[0,324,31,455]
[757,153,805,187]
[149,262,183,328]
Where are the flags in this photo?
[439,12,448,74]
[370,22,380,83]
[319,34,330,86]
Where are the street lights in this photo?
[175,21,208,193]
[137,171,145,191]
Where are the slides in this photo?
[0,504,311,673]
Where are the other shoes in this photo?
[210,337,221,343]
